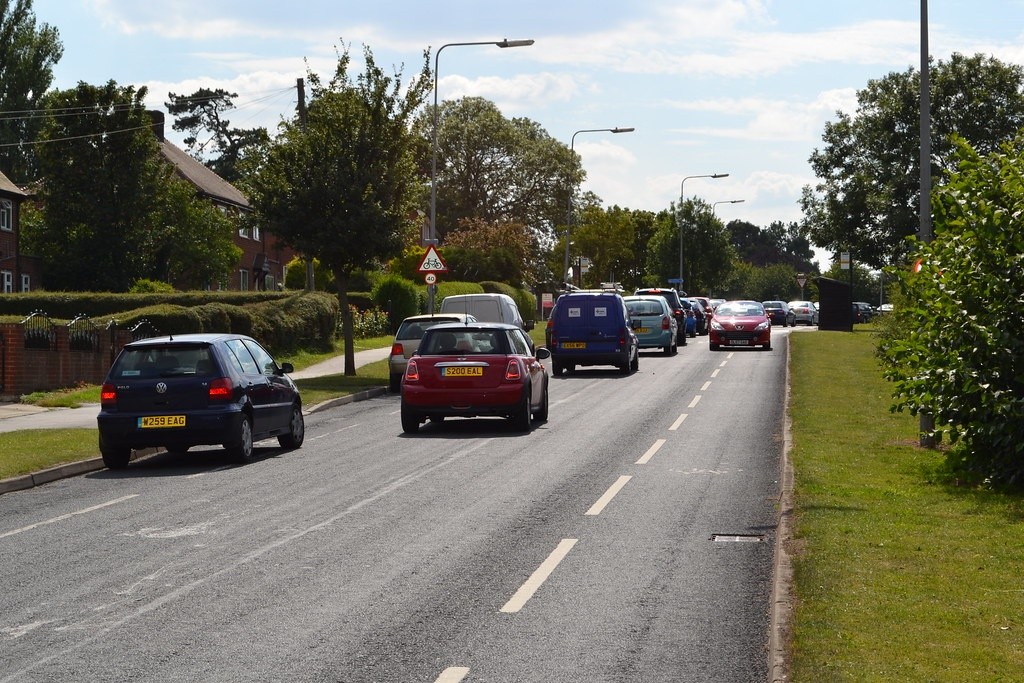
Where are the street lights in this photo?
[427,39,534,316]
[677,173,730,293]
[713,200,745,218]
[560,127,637,288]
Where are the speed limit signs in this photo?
[424,273,436,285]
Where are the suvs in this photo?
[635,288,687,346]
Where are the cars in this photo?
[876,304,894,316]
[681,299,697,338]
[689,297,713,329]
[709,301,771,351]
[623,295,678,358]
[691,300,709,337]
[762,301,797,328]
[788,301,819,326]
[389,311,477,393]
[99,336,305,471]
[401,319,550,435]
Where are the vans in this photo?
[438,293,535,335]
[545,293,640,375]
[852,302,872,325]
